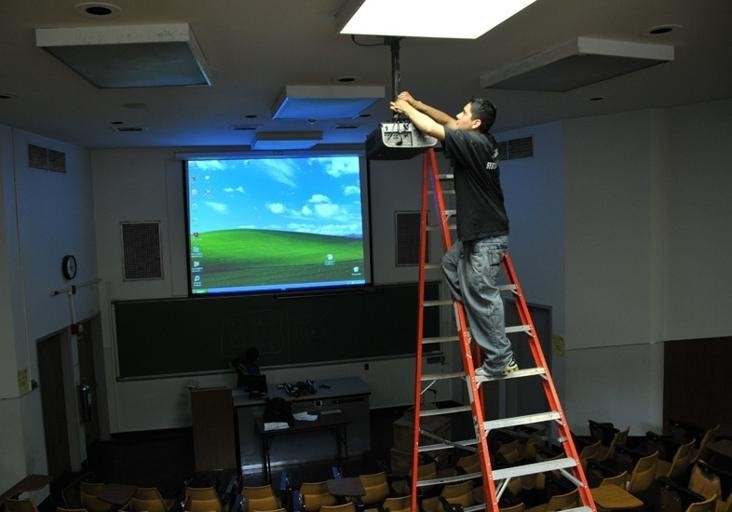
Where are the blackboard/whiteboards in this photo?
[111,279,443,382]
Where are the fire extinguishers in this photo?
[79,382,92,423]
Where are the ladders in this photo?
[411,147,597,512]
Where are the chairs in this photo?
[0,429,732,512]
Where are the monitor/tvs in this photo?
[243,374,268,394]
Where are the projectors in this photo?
[363,121,440,161]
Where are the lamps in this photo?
[251,131,323,151]
[269,85,386,121]
[482,38,675,93]
[35,24,214,88]
[334,0,537,39]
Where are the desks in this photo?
[191,386,237,473]
[256,409,352,483]
[231,376,372,471]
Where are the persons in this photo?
[388,90,520,377]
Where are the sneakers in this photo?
[475,355,518,377]
